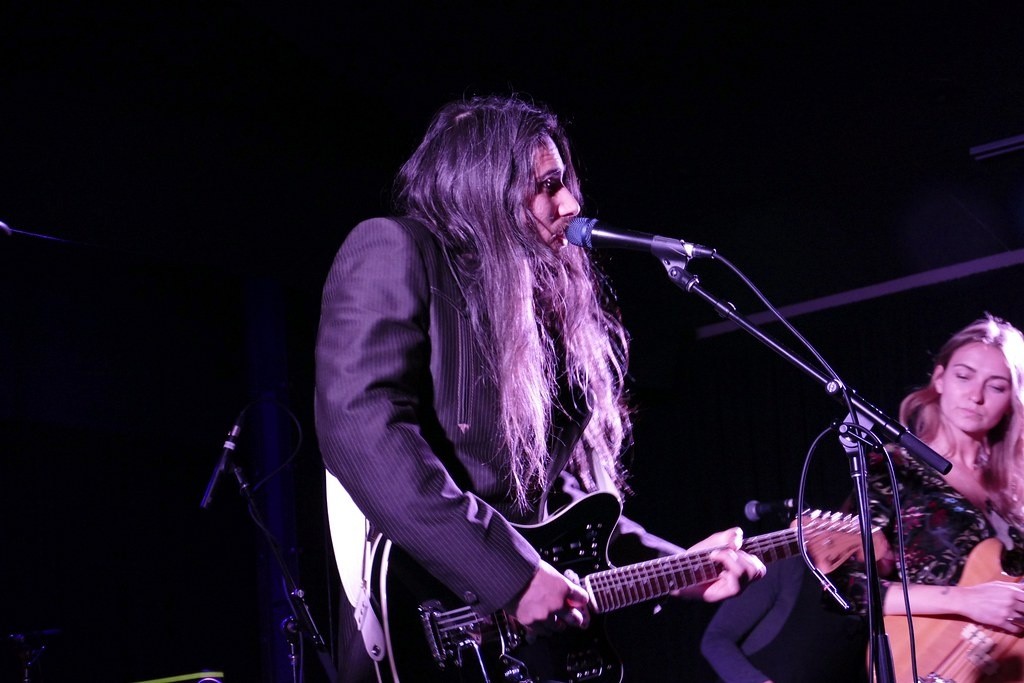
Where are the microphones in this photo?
[200,414,242,509]
[565,217,713,258]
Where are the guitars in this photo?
[359,487,886,682]
[866,537,1023,681]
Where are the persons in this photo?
[312,100,770,683]
[696,475,870,683]
[838,309,1024,682]
